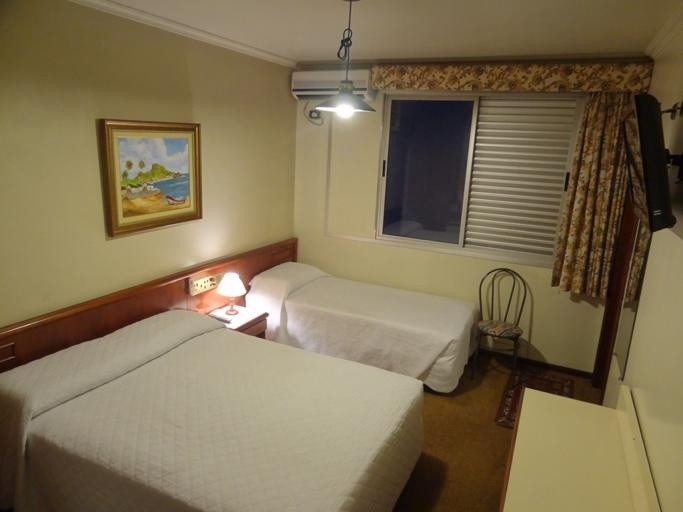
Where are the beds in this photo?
[248,261,478,393]
[1,309,425,512]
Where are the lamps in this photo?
[315,81,375,119]
[216,272,247,316]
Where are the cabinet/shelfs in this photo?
[502,383,664,511]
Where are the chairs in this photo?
[466,268,528,386]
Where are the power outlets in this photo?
[189,274,219,297]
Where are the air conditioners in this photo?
[290,70,376,102]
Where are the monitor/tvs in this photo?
[632,92,676,230]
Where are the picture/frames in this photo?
[96,117,202,239]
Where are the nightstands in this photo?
[206,302,268,340]
